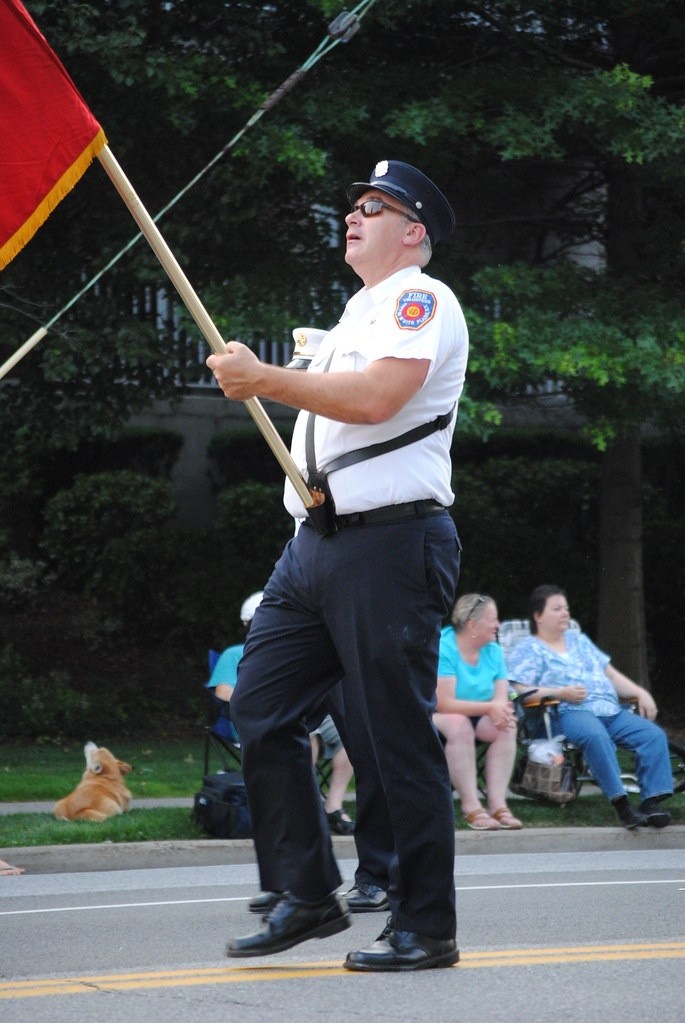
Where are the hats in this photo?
[240,590,263,621]
[285,327,330,370]
[347,160,455,247]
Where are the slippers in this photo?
[493,808,522,830]
[463,809,498,830]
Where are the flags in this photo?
[0,0,108,275]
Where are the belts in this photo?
[301,499,445,528]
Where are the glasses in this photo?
[467,596,485,620]
[350,200,421,223]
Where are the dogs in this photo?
[53,740,132,823]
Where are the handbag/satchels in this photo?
[195,771,252,839]
[510,695,578,804]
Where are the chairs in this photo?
[439,738,488,804]
[498,618,641,804]
[202,648,334,799]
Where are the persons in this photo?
[206,591,356,835]
[507,584,673,829]
[432,594,523,830]
[205,160,472,970]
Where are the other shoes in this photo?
[323,809,355,836]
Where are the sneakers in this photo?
[639,798,672,828]
[614,796,644,829]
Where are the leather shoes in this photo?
[343,884,390,913]
[226,893,352,958]
[343,916,460,972]
[250,891,288,913]
